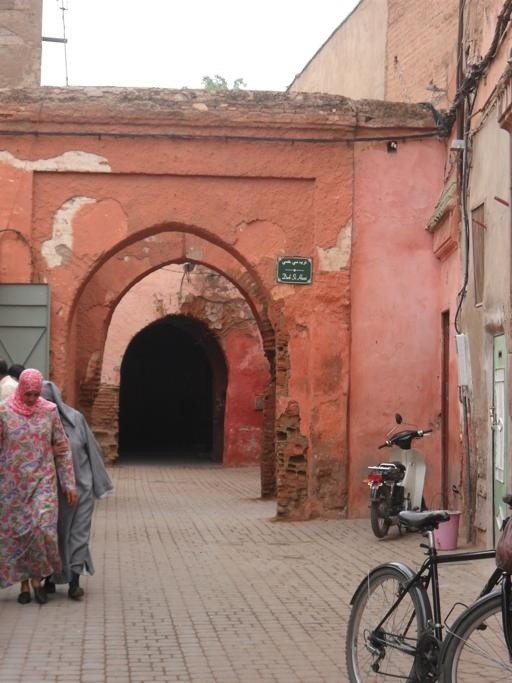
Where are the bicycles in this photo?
[342,493,510,681]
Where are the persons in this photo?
[0,358,20,406]
[6,362,26,382]
[30,379,116,601]
[0,366,80,605]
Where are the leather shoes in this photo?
[18,579,84,603]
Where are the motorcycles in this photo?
[363,411,436,540]
